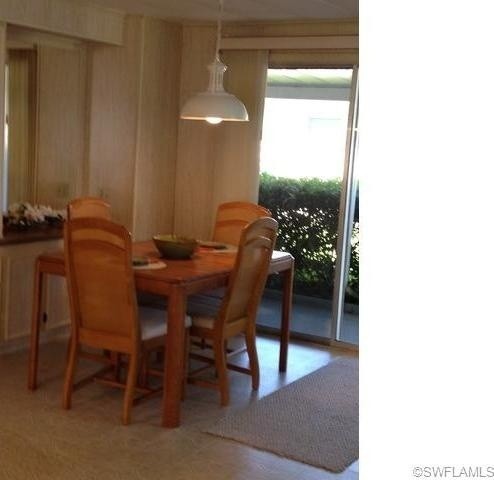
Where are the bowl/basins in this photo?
[152,233,198,260]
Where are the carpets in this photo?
[200,350,359,473]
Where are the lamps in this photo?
[179,0,248,124]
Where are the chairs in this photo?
[63,216,191,426]
[67,197,112,220]
[191,217,278,407]
[211,202,272,247]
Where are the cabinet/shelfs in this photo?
[0,247,72,342]
